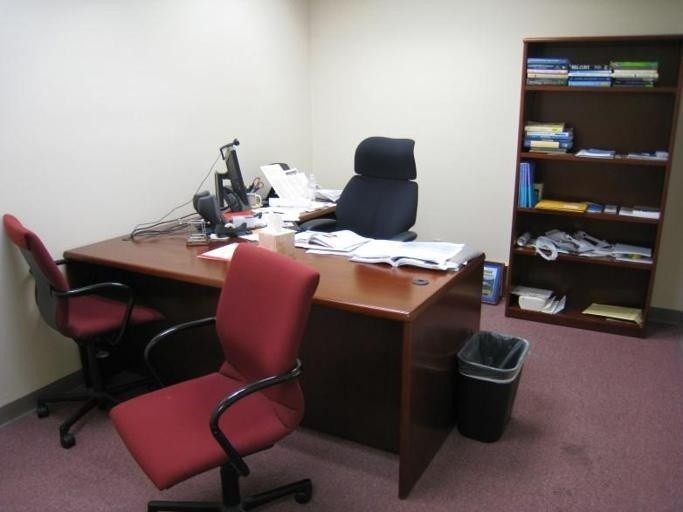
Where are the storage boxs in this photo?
[479,259,505,306]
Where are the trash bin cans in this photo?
[457,330,530,444]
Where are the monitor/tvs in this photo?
[215,144,249,211]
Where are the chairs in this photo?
[1,208,169,450]
[108,240,325,512]
[297,135,422,243]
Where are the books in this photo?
[517,161,536,209]
[522,119,575,154]
[526,57,660,88]
[294,229,482,272]
[615,252,654,264]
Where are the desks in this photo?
[62,191,490,504]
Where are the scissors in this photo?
[249,177,264,192]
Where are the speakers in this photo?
[193,190,210,210]
[197,196,220,222]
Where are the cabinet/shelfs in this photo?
[503,33,681,343]
